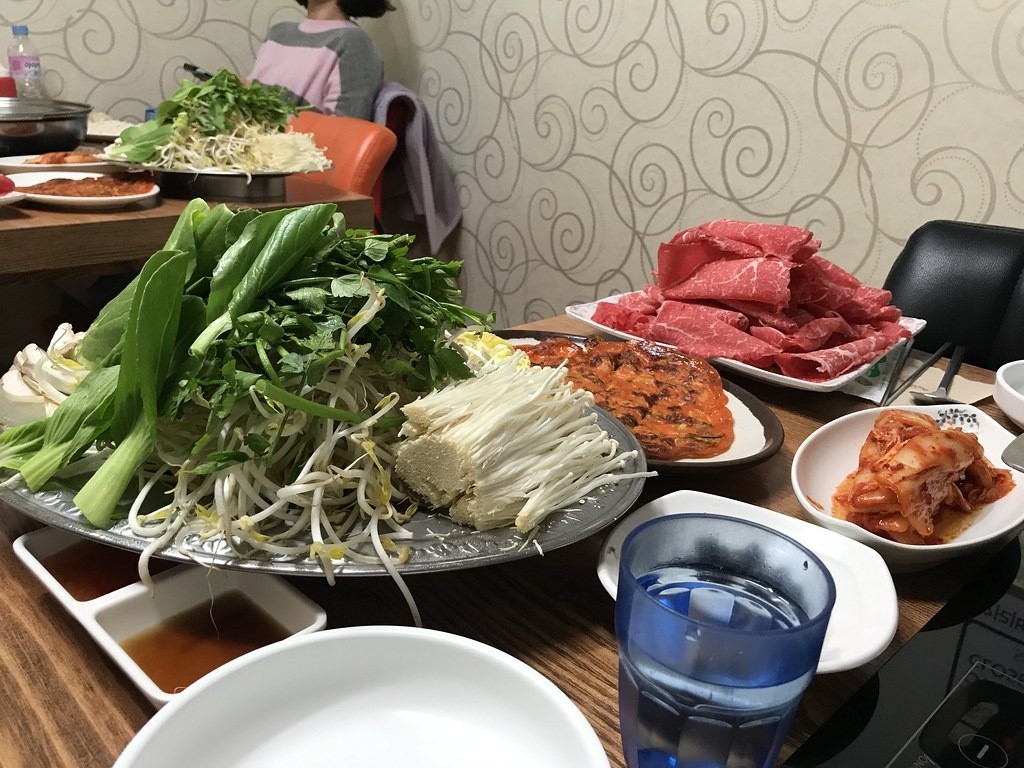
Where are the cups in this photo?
[616,513,836,768]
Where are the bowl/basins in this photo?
[993,360,1024,431]
[0,97,94,157]
[113,626,610,768]
[791,403,1024,573]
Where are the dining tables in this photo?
[0,142,375,306]
[0,312,1024,768]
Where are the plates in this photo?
[13,527,327,711]
[6,171,160,210]
[0,191,27,207]
[597,489,898,674]
[0,155,129,175]
[475,330,784,471]
[565,290,927,392]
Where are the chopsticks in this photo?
[879,338,952,407]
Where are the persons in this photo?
[240,0,397,122]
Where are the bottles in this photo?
[7,25,43,98]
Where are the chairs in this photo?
[880,219,1024,696]
[285,109,397,232]
[371,80,465,256]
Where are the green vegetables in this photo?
[109,67,315,162]
[1,196,496,531]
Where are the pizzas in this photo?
[505,335,734,462]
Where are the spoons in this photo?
[910,343,967,403]
